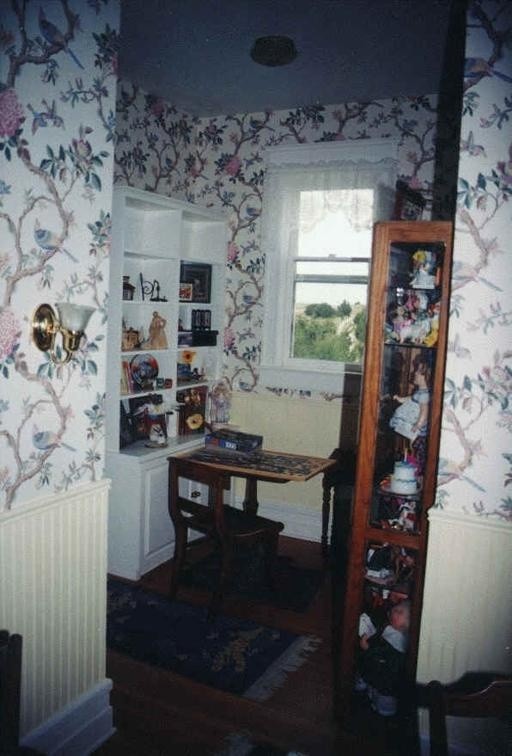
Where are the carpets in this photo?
[177,546,324,613]
[107,572,325,706]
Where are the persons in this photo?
[352,591,414,720]
[363,245,442,588]
[141,311,168,349]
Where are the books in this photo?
[204,427,263,455]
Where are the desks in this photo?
[177,448,331,574]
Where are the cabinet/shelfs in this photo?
[333,219,452,730]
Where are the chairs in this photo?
[166,454,285,623]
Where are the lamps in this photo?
[33,300,95,366]
